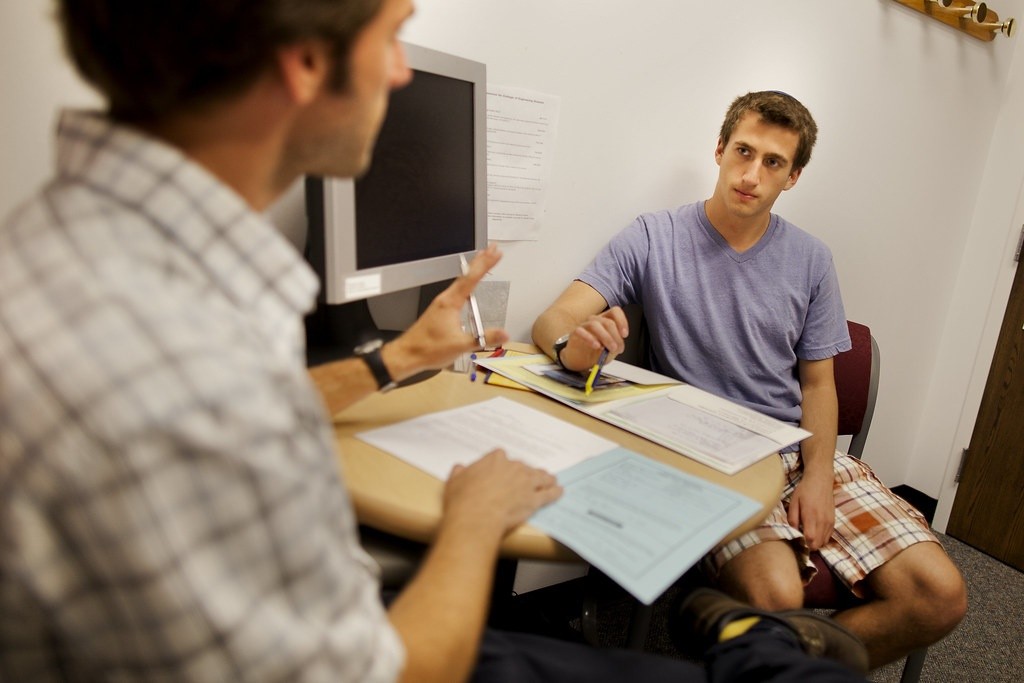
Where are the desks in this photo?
[338,342,789,626]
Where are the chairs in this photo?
[628,320,927,683]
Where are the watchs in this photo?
[554,334,579,374]
[353,338,398,394]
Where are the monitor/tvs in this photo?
[301,41,487,389]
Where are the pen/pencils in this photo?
[458,253,487,349]
[470,352,477,382]
[476,348,505,369]
[585,348,609,396]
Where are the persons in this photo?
[532,89,970,671]
[0,0,872,683]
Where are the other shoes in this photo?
[673,589,870,677]
